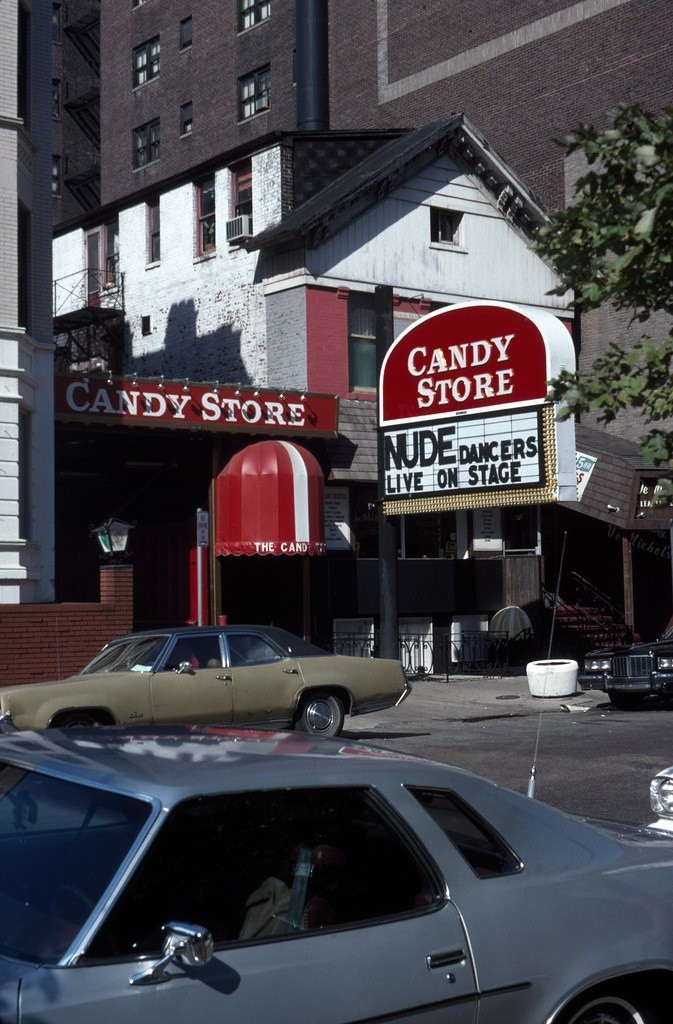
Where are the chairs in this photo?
[206,658,221,667]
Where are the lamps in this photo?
[88,518,138,565]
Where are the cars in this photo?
[1,624,413,738]
[0,725,671,1024]
[578,626,673,707]
